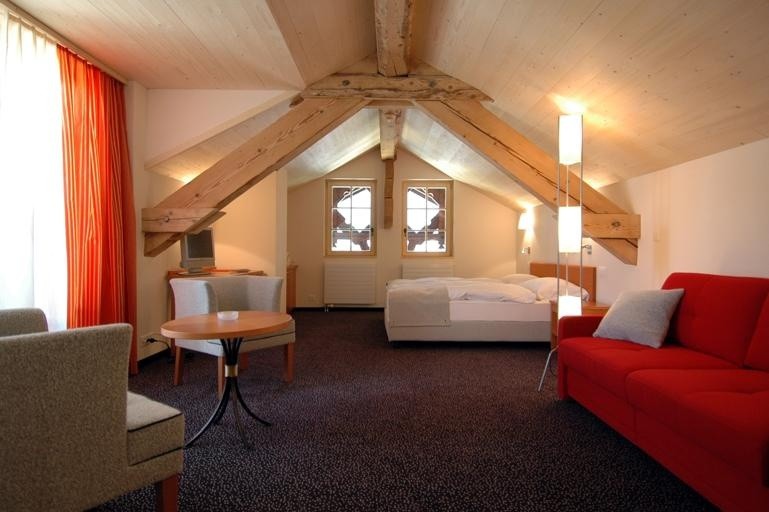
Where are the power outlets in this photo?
[139,331,155,348]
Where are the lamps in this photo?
[558,114,583,319]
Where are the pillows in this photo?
[591,288,686,350]
[521,277,591,303]
[501,273,539,288]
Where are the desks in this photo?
[164,269,266,369]
[286,265,298,314]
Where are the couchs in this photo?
[169,274,299,401]
[556,272,769,511]
[0,306,187,512]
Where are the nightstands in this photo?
[549,301,611,353]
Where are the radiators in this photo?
[401,262,453,280]
[322,261,376,313]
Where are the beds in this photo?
[382,262,597,350]
